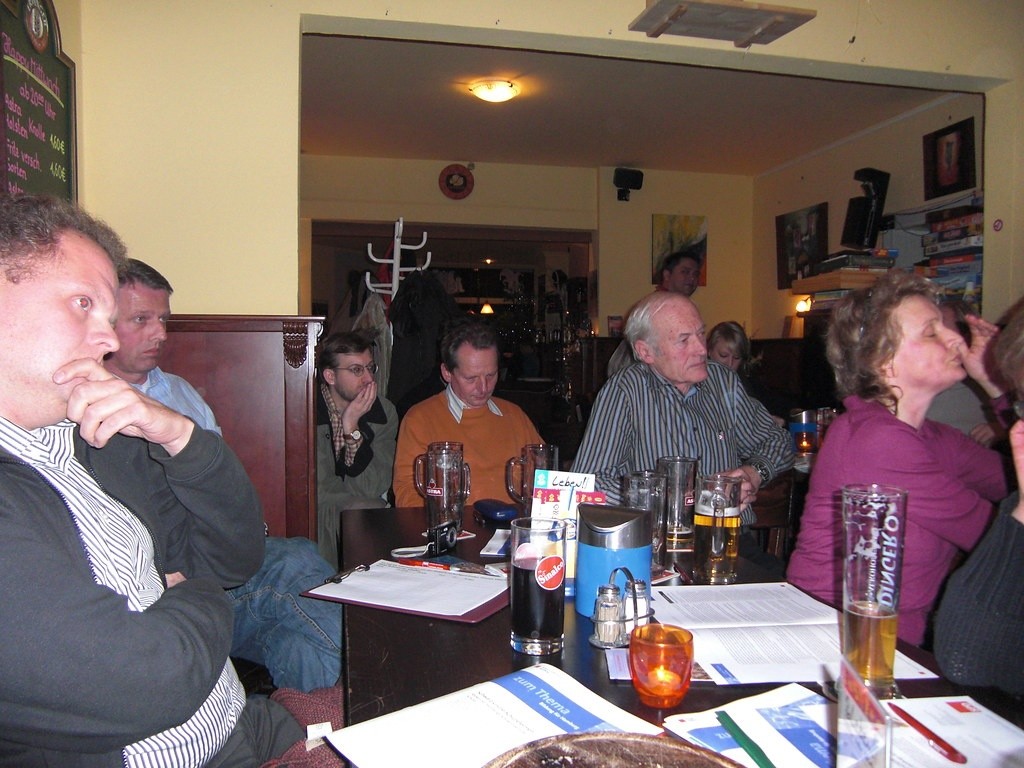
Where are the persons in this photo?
[924,295,1024,717]
[657,250,701,298]
[102,259,346,692]
[705,321,787,426]
[782,266,1018,648]
[568,290,799,580]
[0,199,309,768]
[391,326,547,511]
[315,332,400,575]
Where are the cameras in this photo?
[427,519,458,558]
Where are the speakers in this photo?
[614,168,643,188]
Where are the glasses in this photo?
[328,359,378,377]
[1014,401,1024,420]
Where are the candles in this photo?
[627,622,694,707]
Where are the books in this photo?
[792,204,984,297]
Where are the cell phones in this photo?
[474,498,518,521]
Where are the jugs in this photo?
[790,409,816,450]
[574,502,652,618]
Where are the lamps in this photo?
[612,167,644,201]
[479,299,494,314]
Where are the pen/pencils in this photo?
[887,701,968,763]
[396,558,461,571]
[672,562,696,586]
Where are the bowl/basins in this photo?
[517,377,555,392]
[481,732,748,768]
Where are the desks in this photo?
[339,503,1024,768]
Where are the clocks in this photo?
[439,164,475,200]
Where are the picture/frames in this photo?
[922,116,977,201]
[776,202,828,290]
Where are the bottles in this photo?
[622,579,650,641]
[540,295,563,328]
[543,342,578,403]
[595,584,622,643]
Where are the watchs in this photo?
[343,430,362,441]
[744,460,769,486]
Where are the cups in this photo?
[506,444,558,517]
[817,409,838,450]
[509,517,566,656]
[623,471,667,576]
[693,474,742,585]
[414,441,470,534]
[794,432,816,453]
[656,456,698,550]
[835,482,908,704]
[629,623,695,708]
[545,319,592,342]
